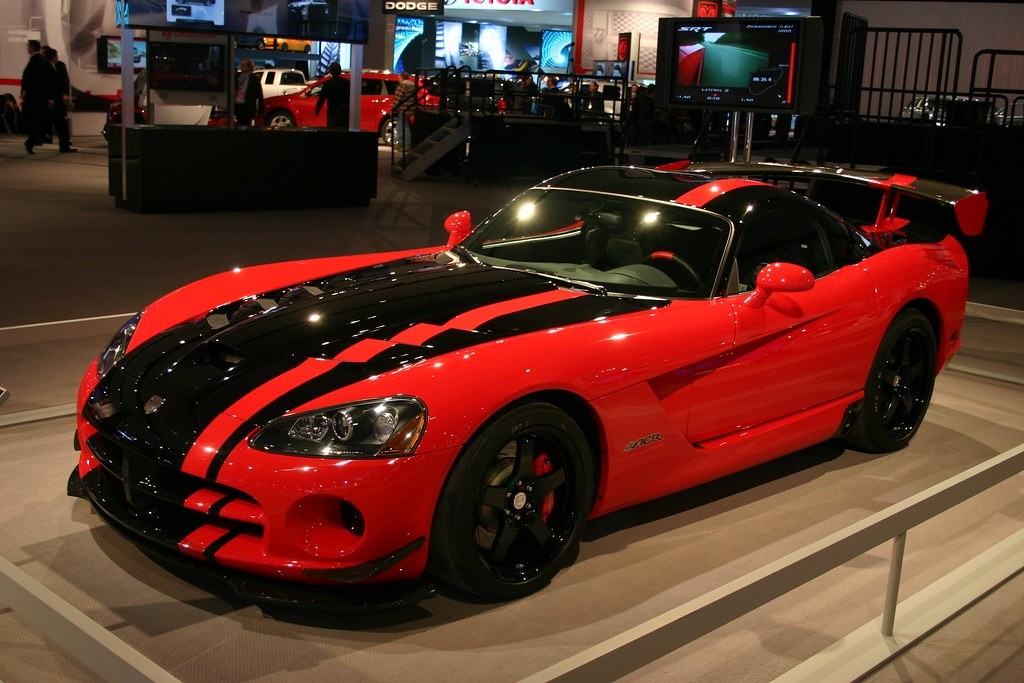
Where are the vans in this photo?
[252,69,306,99]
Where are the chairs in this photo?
[727,210,835,286]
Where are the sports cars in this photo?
[68,157,985,606]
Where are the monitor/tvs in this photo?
[146,41,227,92]
[654,17,826,115]
[101,35,147,75]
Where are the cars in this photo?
[101,70,237,144]
[288,0,330,16]
[134,47,140,62]
[234,36,266,50]
[264,37,312,54]
[903,96,993,127]
[257,73,439,147]
[988,102,1024,127]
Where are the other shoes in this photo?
[25,140,34,155]
[59,148,78,153]
[34,141,42,146]
[68,141,73,146]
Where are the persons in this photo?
[234,58,265,125]
[315,62,352,128]
[390,71,416,153]
[516,74,655,147]
[21,40,77,154]
